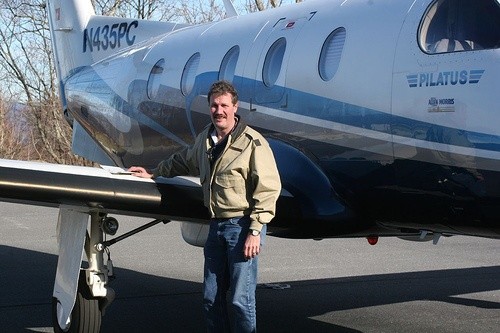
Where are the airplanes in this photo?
[0,0,500,333]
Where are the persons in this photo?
[127,80,281,333]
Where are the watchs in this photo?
[249,228,260,236]
